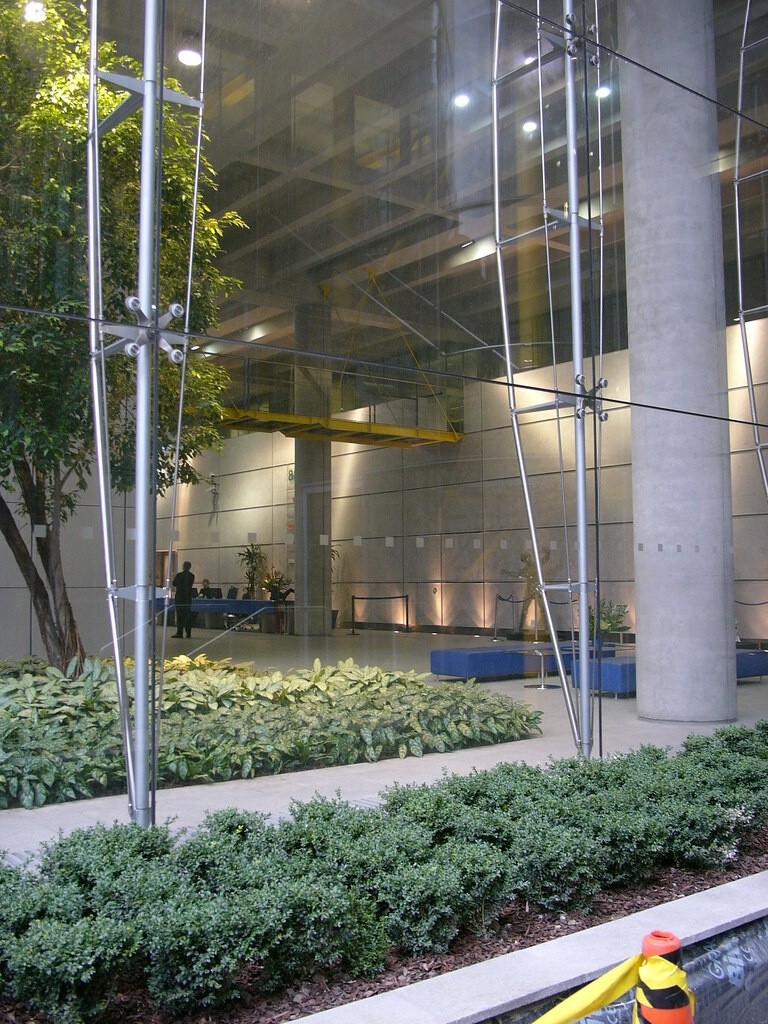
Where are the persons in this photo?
[171,561,195,638]
[191,579,210,628]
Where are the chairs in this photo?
[241,586,252,598]
[226,587,238,599]
[190,588,197,598]
[211,588,222,598]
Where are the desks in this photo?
[155,598,275,633]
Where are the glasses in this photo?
[202,583,207,585]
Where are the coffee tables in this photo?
[562,646,632,658]
[510,649,578,690]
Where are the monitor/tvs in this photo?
[210,588,222,599]
[191,588,198,599]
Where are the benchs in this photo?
[569,649,767,699]
[430,640,616,681]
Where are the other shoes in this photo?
[171,633,182,638]
[187,633,190,638]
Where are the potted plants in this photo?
[261,570,295,635]
